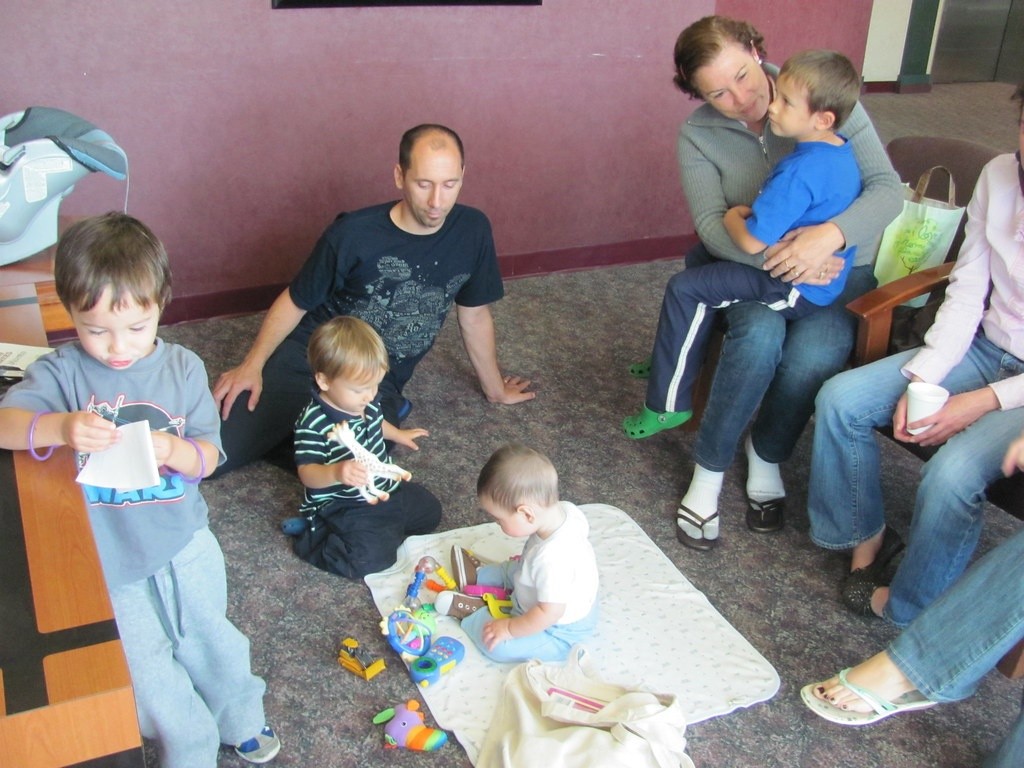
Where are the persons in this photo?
[673,14,906,556]
[279,315,443,581]
[806,73,1024,632]
[433,443,601,664]
[798,431,1024,768]
[620,50,862,441]
[203,124,536,481]
[0,210,283,768]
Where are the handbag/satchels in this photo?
[873,165,967,309]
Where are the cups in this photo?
[907,383,949,435]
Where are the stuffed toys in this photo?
[372,699,448,751]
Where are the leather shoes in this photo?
[842,580,892,620]
[845,523,906,585]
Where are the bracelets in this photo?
[179,436,206,484]
[28,411,54,463]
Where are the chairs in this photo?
[680,135,1024,679]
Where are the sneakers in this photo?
[434,590,488,621]
[234,722,281,764]
[451,545,481,593]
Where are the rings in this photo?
[505,380,508,383]
[819,272,824,280]
[785,261,791,269]
[826,262,833,274]
[791,270,800,277]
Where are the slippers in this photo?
[629,356,650,378]
[746,496,785,532]
[622,399,694,439]
[280,517,307,535]
[800,667,939,726]
[676,473,720,550]
